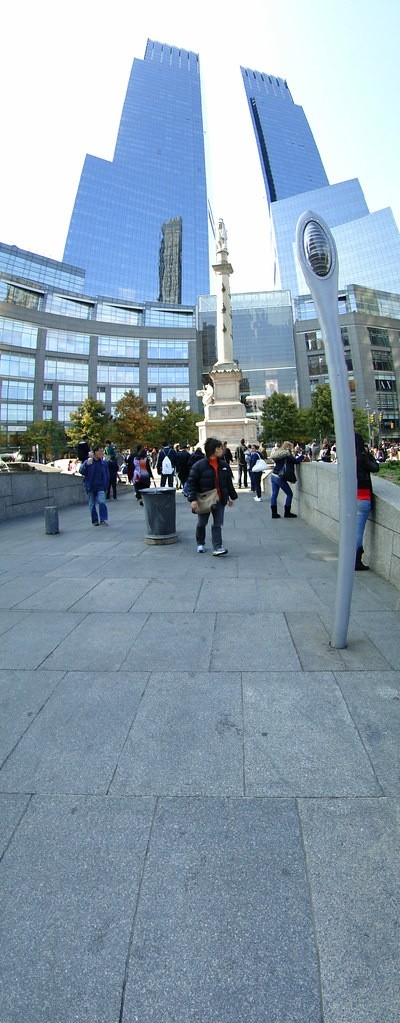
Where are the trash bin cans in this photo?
[139,487,178,545]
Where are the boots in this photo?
[355,549,370,570]
[284,505,297,518]
[271,505,282,518]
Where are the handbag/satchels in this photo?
[278,458,297,484]
[132,461,141,484]
[191,488,220,515]
[251,453,267,472]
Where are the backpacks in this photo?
[161,448,174,474]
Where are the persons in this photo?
[365,441,400,462]
[104,439,116,461]
[223,439,281,502]
[79,445,110,527]
[187,438,238,555]
[117,445,157,485]
[354,432,380,571]
[77,436,90,464]
[294,438,337,463]
[156,442,204,497]
[271,441,306,519]
[68,457,81,472]
[106,455,120,500]
[133,449,154,506]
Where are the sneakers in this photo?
[197,545,205,552]
[212,547,228,556]
[254,495,261,501]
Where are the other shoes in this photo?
[100,521,108,526]
[92,522,99,526]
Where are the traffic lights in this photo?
[370,414,374,422]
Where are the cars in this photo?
[0,453,76,473]
